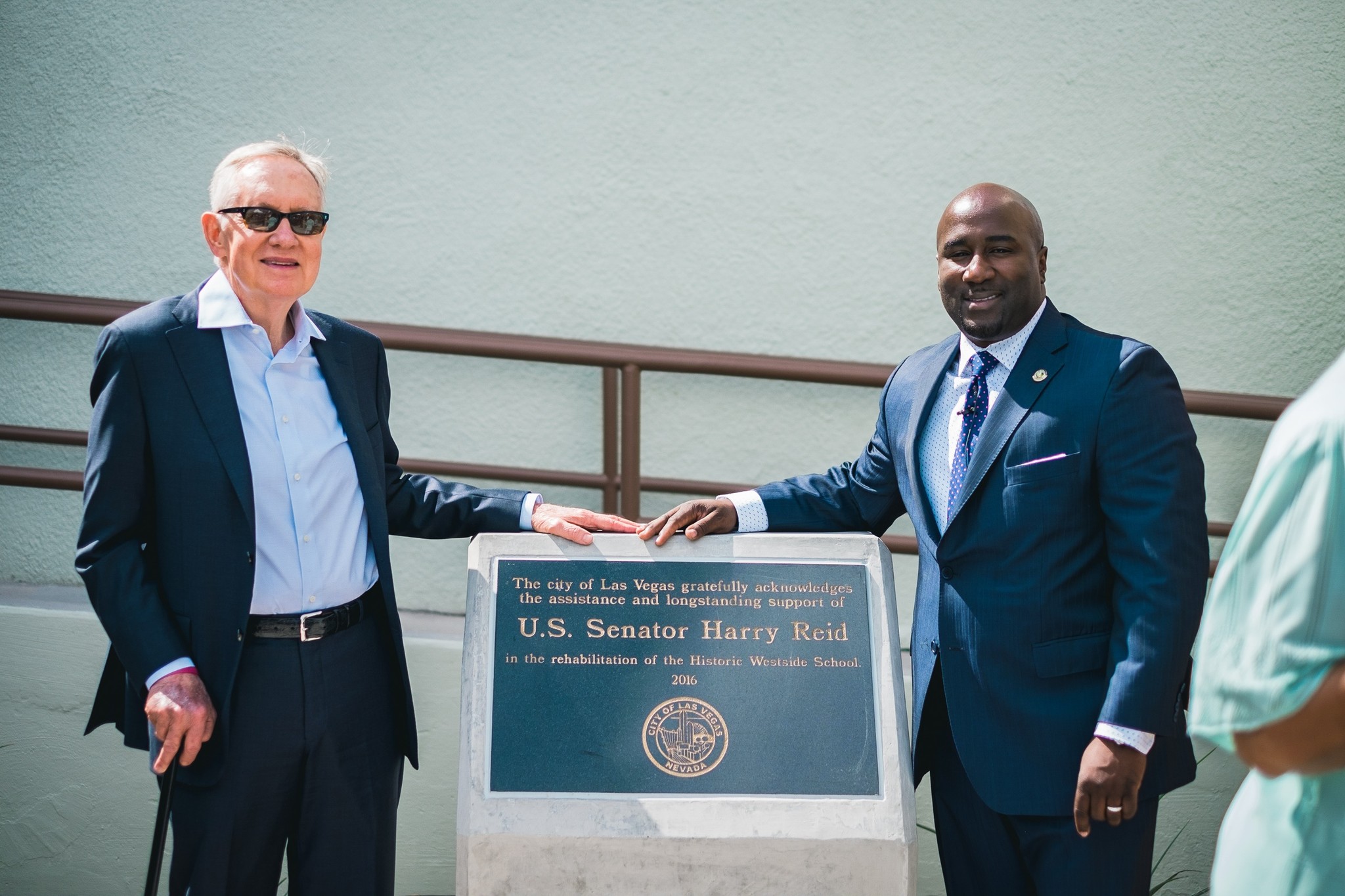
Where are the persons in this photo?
[75,138,643,896]
[1186,352,1344,896]
[638,183,1209,896]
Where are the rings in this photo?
[1105,805,1124,812]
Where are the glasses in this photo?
[217,206,329,235]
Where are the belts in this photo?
[246,594,375,642]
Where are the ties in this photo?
[947,352,1000,523]
